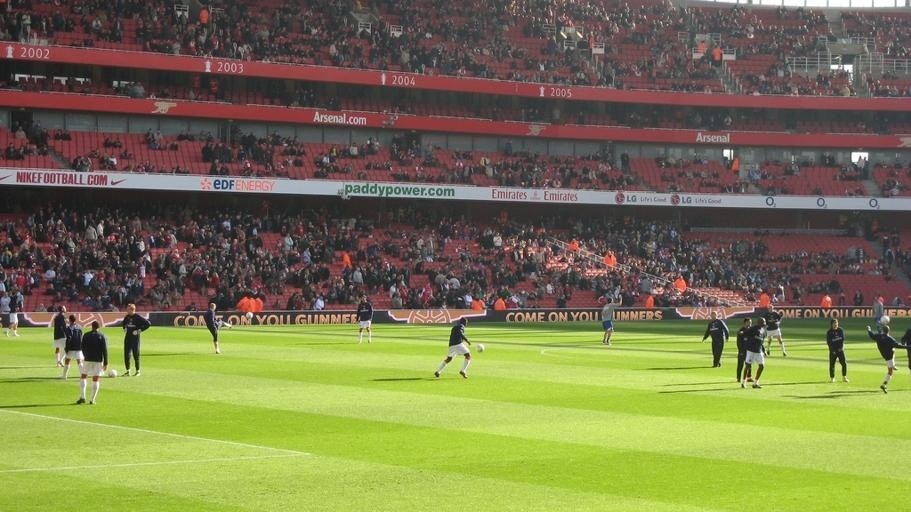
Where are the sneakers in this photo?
[830,376,888,394]
[433,370,468,378]
[4,330,20,337]
[603,339,611,345]
[737,378,764,389]
[76,397,97,404]
[57,360,64,367]
[766,350,789,357]
[122,369,140,377]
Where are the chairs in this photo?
[3,0,911,313]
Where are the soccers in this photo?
[880,315,890,325]
[110,370,117,377]
[246,312,253,319]
[477,344,484,352]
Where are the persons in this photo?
[52,305,69,368]
[601,294,624,346]
[77,321,110,405]
[356,293,375,346]
[871,294,884,325]
[121,302,152,377]
[3,291,21,338]
[867,324,911,393]
[62,315,86,381]
[434,317,472,379]
[823,318,849,382]
[763,304,789,357]
[735,317,754,382]
[700,310,731,368]
[902,317,911,370]
[204,302,233,355]
[740,318,768,389]
